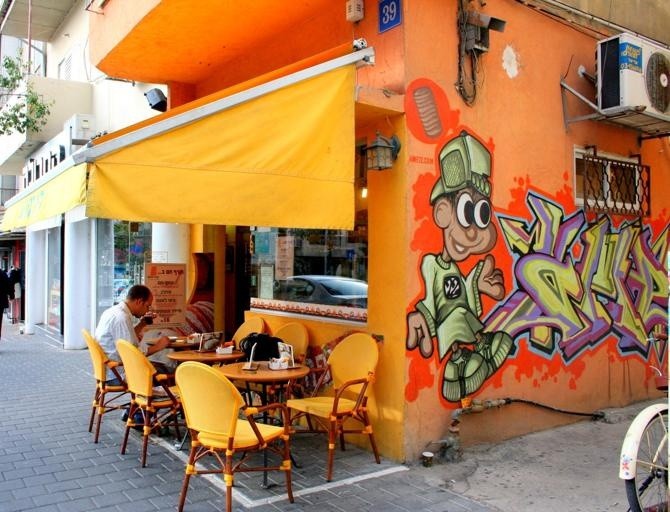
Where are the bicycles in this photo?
[616,324,670,512]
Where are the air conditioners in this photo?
[594,33,670,135]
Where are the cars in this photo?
[273,273,367,311]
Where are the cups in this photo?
[144,312,154,325]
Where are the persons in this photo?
[95,284,178,434]
[10,266,23,300]
[1,267,16,346]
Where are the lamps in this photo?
[363,131,401,171]
[144,88,167,112]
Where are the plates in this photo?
[167,336,178,342]
